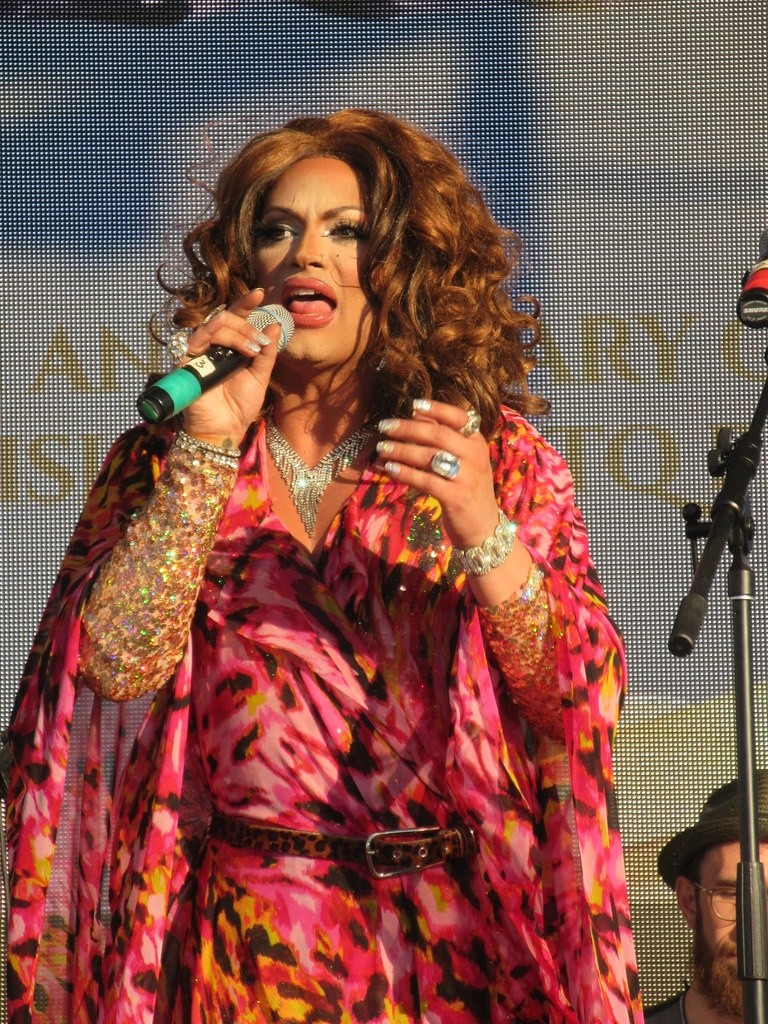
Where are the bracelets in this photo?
[176,430,245,470]
[452,510,519,575]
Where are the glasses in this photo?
[692,881,738,921]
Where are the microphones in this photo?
[135,303,295,426]
[736,230,767,330]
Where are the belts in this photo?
[209,813,478,879]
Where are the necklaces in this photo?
[263,415,377,539]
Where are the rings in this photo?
[204,303,227,324]
[459,407,481,439]
[430,452,463,482]
[164,332,200,361]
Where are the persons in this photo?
[0,109,644,1024]
[643,771,768,1023]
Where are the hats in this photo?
[656,768,768,892]
